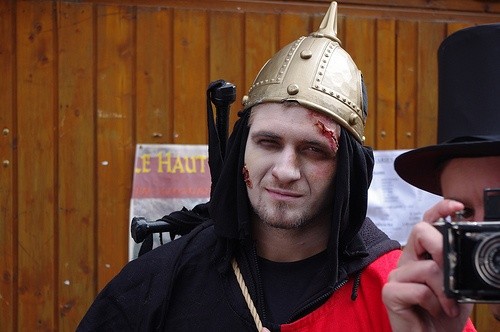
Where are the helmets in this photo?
[241,2,369,145]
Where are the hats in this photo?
[394,25,500,197]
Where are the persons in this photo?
[75,2,479,332]
[382,25,500,332]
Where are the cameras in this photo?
[433,188,500,304]
